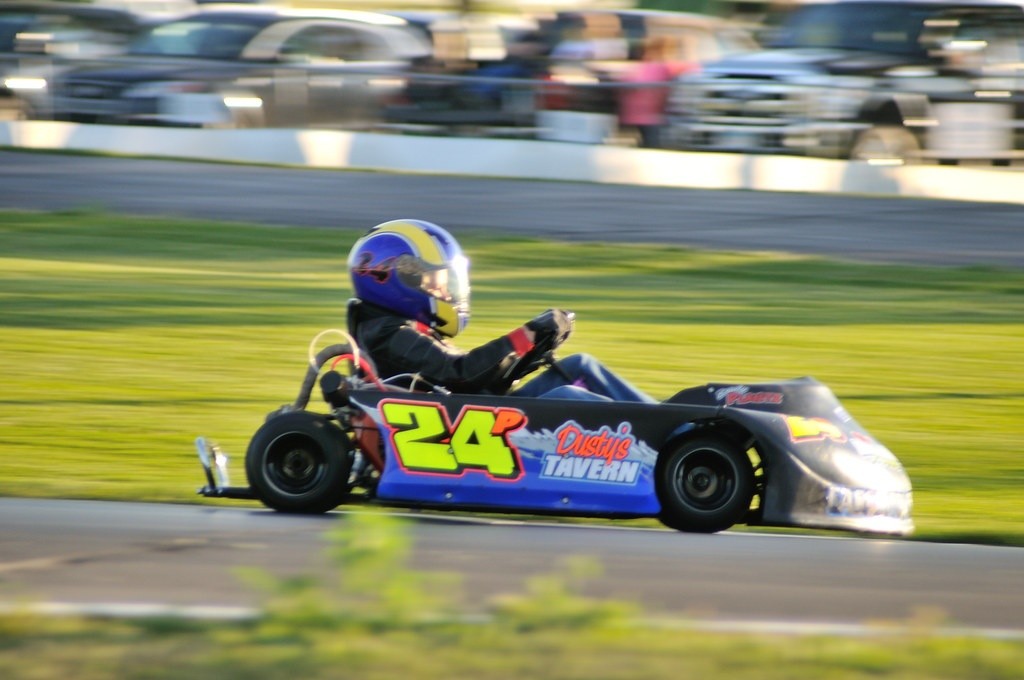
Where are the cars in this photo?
[1,0,1024,166]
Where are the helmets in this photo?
[349,219,471,340]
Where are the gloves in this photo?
[526,307,570,349]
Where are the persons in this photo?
[852,98,921,162]
[347,219,661,406]
[614,35,699,147]
